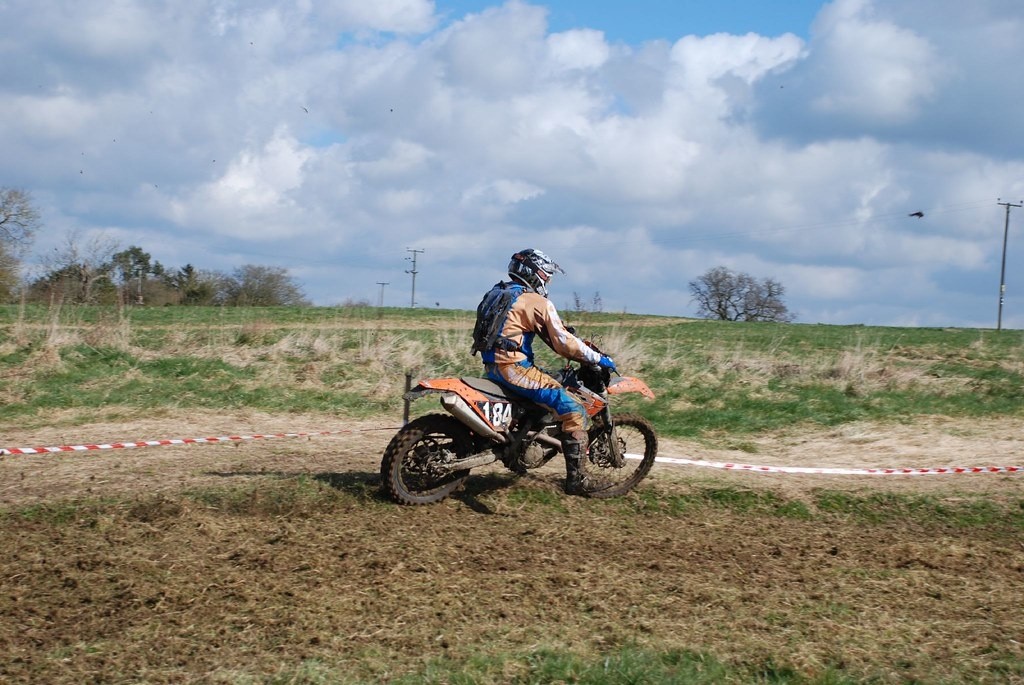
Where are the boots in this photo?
[560,431,609,494]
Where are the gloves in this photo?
[597,355,617,373]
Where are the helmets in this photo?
[507,248,567,297]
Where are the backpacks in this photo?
[469,280,527,356]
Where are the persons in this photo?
[481,248,615,495]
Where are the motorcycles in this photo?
[379,331,659,507]
